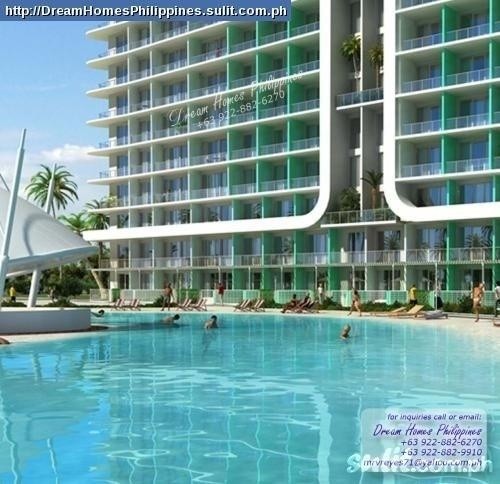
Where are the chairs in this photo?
[176,297,207,311]
[233,299,265,312]
[285,298,319,313]
[370,304,448,320]
[111,299,141,311]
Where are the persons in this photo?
[470,280,484,322]
[161,281,175,311]
[339,324,351,339]
[347,290,361,316]
[280,293,314,313]
[163,314,180,326]
[217,282,224,306]
[9,283,17,302]
[493,280,500,320]
[204,315,218,329]
[410,284,417,305]
[91,309,104,317]
